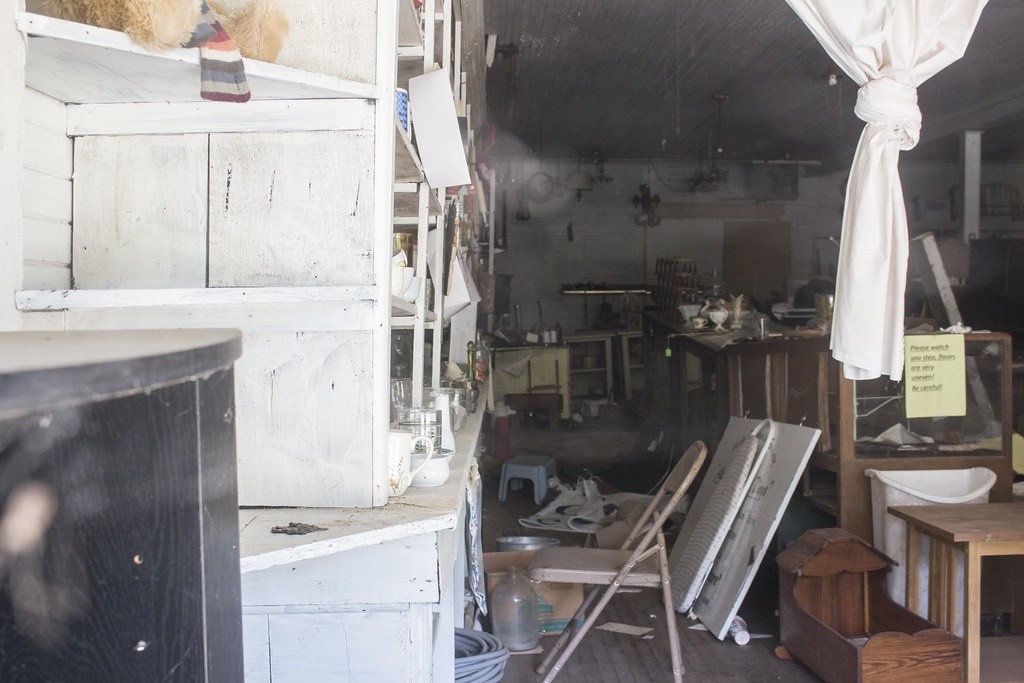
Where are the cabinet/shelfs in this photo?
[612,327,653,402]
[561,329,615,404]
[491,341,573,427]
[0,0,489,683]
[724,329,1013,551]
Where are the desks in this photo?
[0,328,245,683]
[643,306,796,490]
[887,502,1024,683]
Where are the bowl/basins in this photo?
[496,536,560,553]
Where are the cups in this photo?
[728,616,751,645]
[392,231,422,302]
[389,376,474,498]
[692,317,708,328]
[814,292,835,330]
[678,305,701,326]
[710,311,728,330]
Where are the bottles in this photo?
[554,322,562,341]
[464,341,478,412]
[475,331,490,377]
[491,565,540,652]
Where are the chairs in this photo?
[528,440,708,683]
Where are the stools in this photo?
[503,393,564,433]
[498,455,558,505]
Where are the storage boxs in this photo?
[482,550,586,637]
[776,529,965,683]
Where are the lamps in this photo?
[561,282,659,295]
